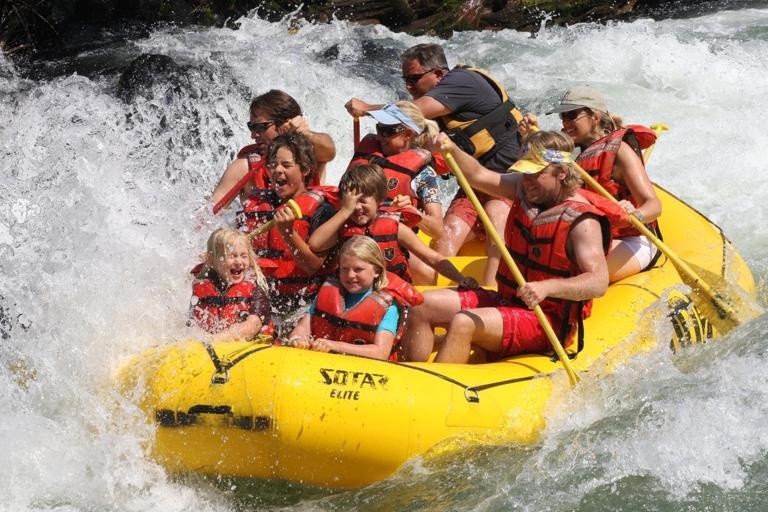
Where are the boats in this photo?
[108,181,758,475]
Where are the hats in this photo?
[506,146,574,175]
[364,101,424,135]
[545,84,608,115]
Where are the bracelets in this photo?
[636,210,644,222]
[287,335,302,346]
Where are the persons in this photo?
[345,43,528,289]
[307,163,480,302]
[186,227,280,347]
[286,234,406,361]
[338,100,444,286]
[518,86,664,285]
[398,131,611,365]
[209,89,336,260]
[190,131,336,314]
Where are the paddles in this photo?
[439,144,586,395]
[524,121,764,336]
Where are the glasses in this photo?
[402,69,436,84]
[247,119,287,132]
[559,109,592,121]
[376,124,408,137]
